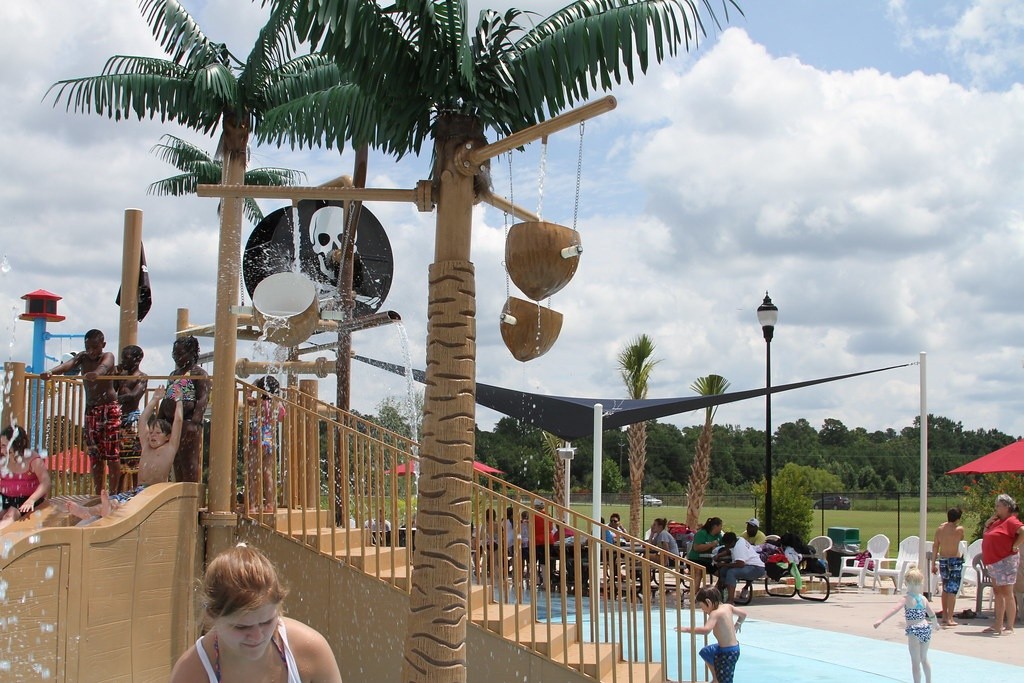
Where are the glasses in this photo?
[610,519,619,523]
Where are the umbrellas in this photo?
[44,447,109,474]
[945,438,1024,475]
[384,455,505,473]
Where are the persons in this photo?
[114,345,148,494]
[66,385,183,520]
[158,335,211,482]
[365,509,390,529]
[982,494,1024,632]
[874,568,940,683]
[644,518,679,566]
[171,542,342,683]
[931,509,965,625]
[0,425,51,531]
[674,584,747,683]
[248,376,285,513]
[480,499,629,584]
[40,329,122,495]
[686,517,765,606]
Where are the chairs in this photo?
[808,536,833,584]
[838,533,994,618]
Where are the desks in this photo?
[518,542,731,602]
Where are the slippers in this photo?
[983,625,1002,633]
[999,628,1014,636]
[935,609,987,620]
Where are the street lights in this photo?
[756,288,779,536]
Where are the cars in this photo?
[814,495,850,510]
[640,494,662,507]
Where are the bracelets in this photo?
[49,371,52,375]
[984,525,988,528]
[1013,547,1019,549]
[174,398,181,401]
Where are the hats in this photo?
[534,499,545,509]
[745,517,760,528]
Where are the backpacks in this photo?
[856,550,874,569]
